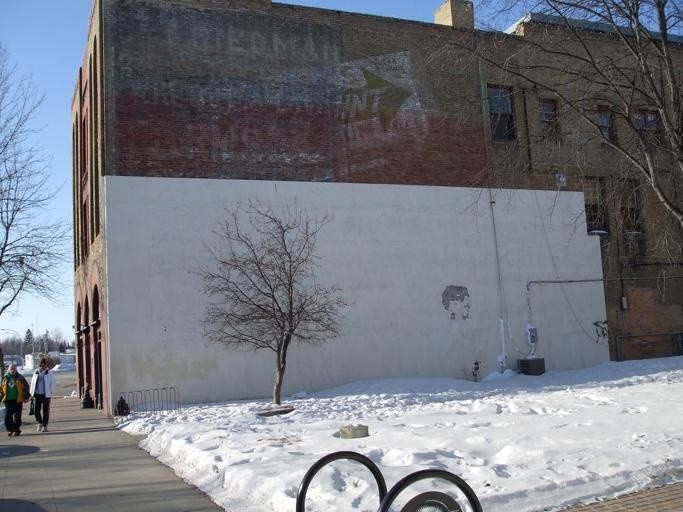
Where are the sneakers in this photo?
[37,423,48,432]
[8,430,21,436]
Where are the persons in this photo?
[1,364,30,437]
[29,358,54,432]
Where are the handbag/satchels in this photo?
[29,398,35,416]
